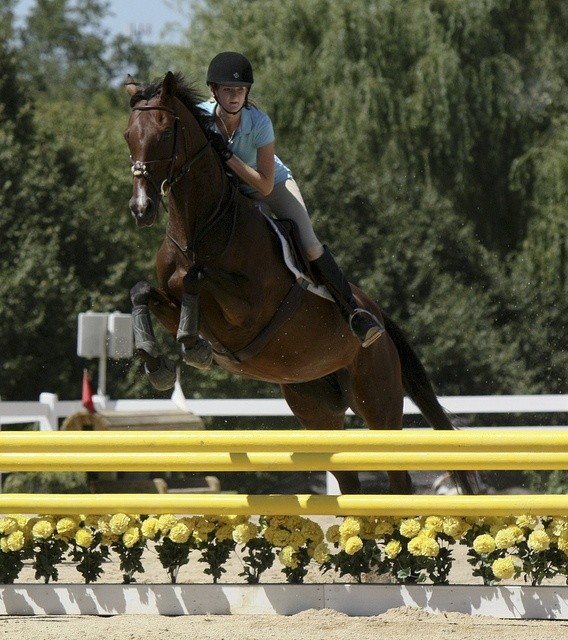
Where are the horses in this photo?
[123,68,484,496]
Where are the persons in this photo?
[190,51,384,348]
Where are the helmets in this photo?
[206,51,254,88]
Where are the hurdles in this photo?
[0,429,568,515]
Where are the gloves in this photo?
[211,132,234,163]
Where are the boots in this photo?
[308,244,381,343]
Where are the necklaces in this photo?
[215,108,238,144]
